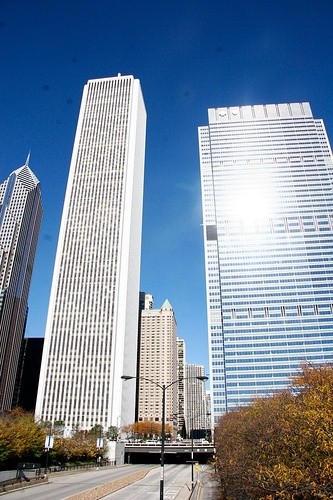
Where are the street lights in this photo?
[121,375,208,499]
[173,412,212,490]
